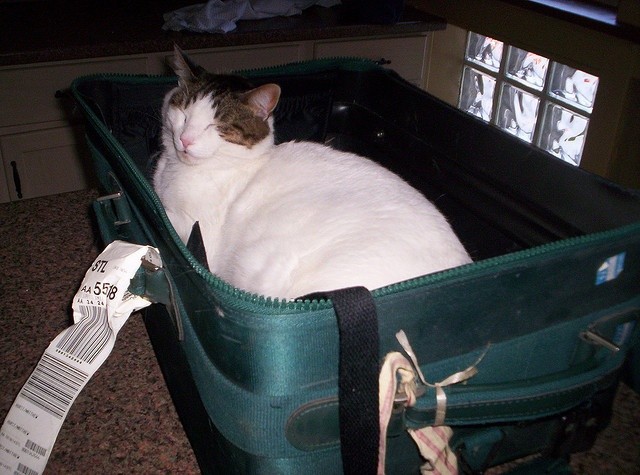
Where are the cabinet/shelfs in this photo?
[0,29,433,203]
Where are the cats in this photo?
[142,41,476,303]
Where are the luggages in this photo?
[55,56,639,474]
